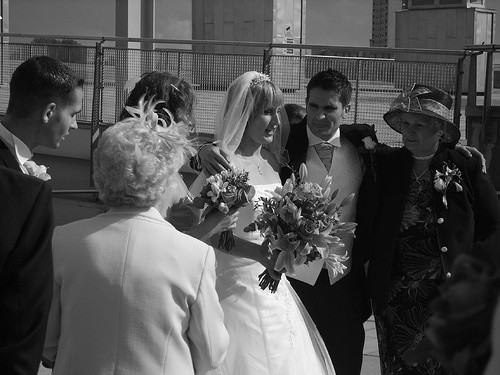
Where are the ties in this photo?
[318,144,333,172]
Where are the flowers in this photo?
[194,166,256,251]
[433,161,463,206]
[245,163,358,294]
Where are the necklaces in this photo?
[244,149,266,176]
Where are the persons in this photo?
[0,55,85,183]
[118,70,241,243]
[366,82,500,375]
[189,68,487,375]
[186,70,337,375]
[0,163,55,375]
[284,103,308,125]
[39,118,231,375]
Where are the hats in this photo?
[382,83,461,147]
